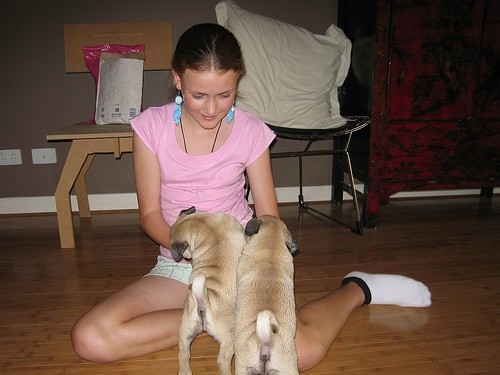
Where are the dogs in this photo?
[235,214,300,375]
[169,206,246,375]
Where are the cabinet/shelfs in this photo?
[330,0,500,230]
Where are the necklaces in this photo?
[179,98,222,154]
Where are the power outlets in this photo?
[0,148,23,166]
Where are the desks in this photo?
[45,121,134,250]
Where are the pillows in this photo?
[214,0,353,128]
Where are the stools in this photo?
[246,115,373,236]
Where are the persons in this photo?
[70,23,432,372]
